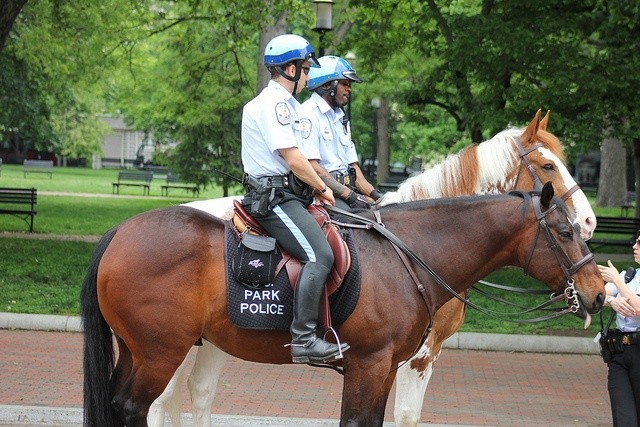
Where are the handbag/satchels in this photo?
[230,227,277,291]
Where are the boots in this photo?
[290,262,351,364]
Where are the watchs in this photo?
[607,296,613,305]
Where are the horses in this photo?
[146,107,598,427]
[81,180,606,427]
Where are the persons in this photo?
[301,56,382,210]
[597,231,640,427]
[241,35,350,364]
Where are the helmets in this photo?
[264,33,321,68]
[307,55,363,90]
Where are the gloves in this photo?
[345,190,371,208]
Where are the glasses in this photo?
[285,64,309,75]
[635,238,640,247]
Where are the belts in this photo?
[622,332,640,345]
[330,171,355,186]
[247,176,289,188]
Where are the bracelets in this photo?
[321,186,327,193]
